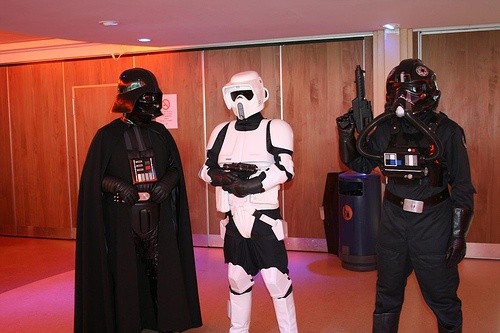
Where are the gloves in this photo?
[444,205,469,269]
[335,113,360,163]
[147,173,186,207]
[205,169,246,186]
[221,178,266,198]
[102,174,140,206]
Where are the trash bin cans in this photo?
[337,170,382,273]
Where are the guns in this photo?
[335,64,374,134]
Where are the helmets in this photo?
[222,71,268,120]
[384,57,442,118]
[112,68,163,116]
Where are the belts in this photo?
[384,188,452,207]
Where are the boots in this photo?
[372,312,399,333]
[271,292,299,333]
[435,311,463,333]
[226,285,251,333]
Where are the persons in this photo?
[198,71,298,333]
[73,68,203,333]
[336,59,474,333]
